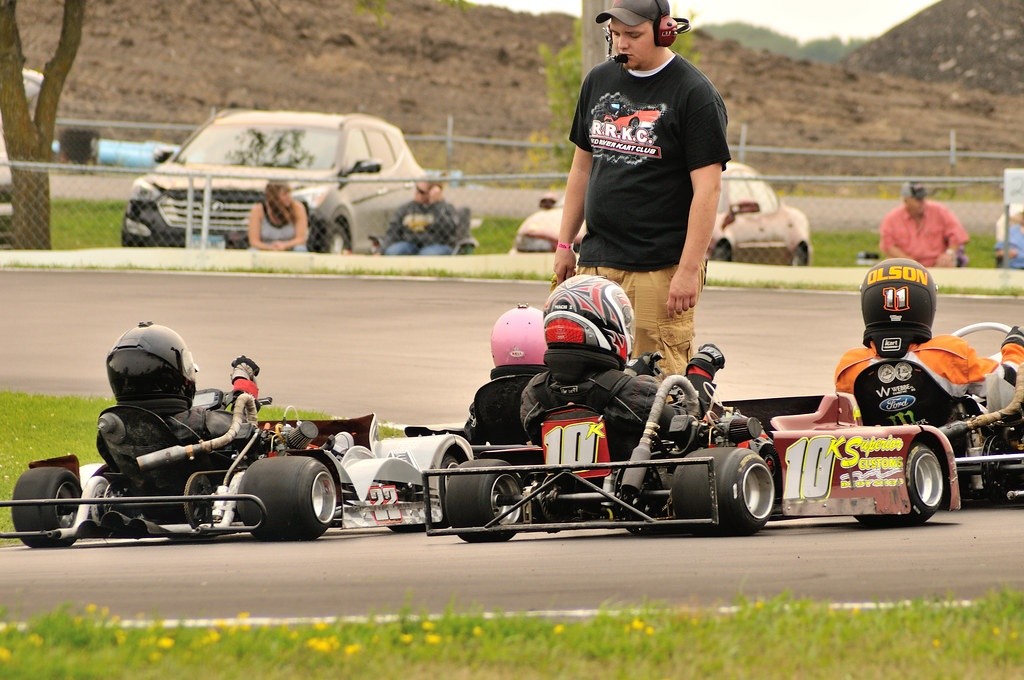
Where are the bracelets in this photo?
[558,243,574,250]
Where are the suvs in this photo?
[122,108,429,255]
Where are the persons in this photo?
[553,0,732,404]
[248,182,307,252]
[995,211,1024,269]
[382,180,463,256]
[834,258,1024,427]
[106,321,261,454]
[879,181,969,268]
[520,275,724,460]
[490,305,549,378]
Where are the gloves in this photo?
[626,352,658,376]
[1000,326,1024,350]
[228,356,262,386]
[683,343,725,378]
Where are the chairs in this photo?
[367,207,478,254]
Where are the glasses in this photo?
[415,185,429,195]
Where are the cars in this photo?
[514,160,813,268]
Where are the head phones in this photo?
[605,0,691,47]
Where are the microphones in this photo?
[607,39,628,63]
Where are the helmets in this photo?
[103,322,202,412]
[535,274,637,370]
[858,257,939,357]
[490,301,548,369]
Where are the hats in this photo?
[594,0,671,30]
[902,183,928,199]
